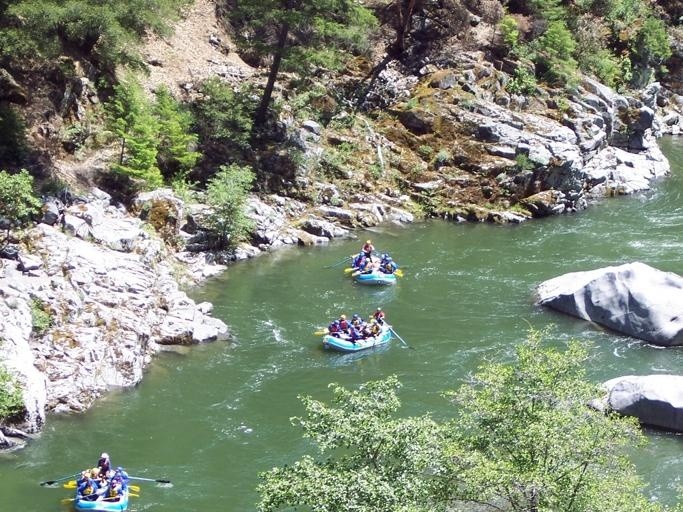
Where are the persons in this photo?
[78,452,130,501]
[353,239,399,274]
[328,308,385,340]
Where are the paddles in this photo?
[314,331,344,337]
[345,267,404,279]
[41,466,171,504]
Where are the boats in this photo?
[322,324,392,351]
[351,254,398,285]
[73,467,128,512]
